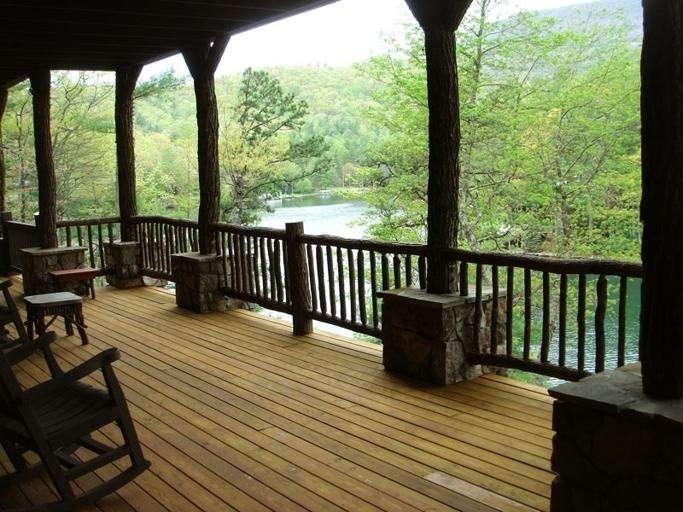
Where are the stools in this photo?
[23,292,88,346]
[48,264,104,300]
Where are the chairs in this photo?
[0,277,28,350]
[0,331,151,512]
